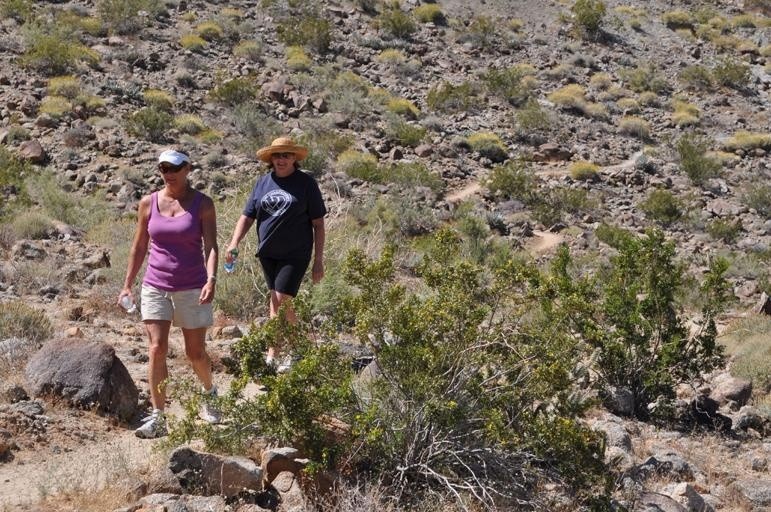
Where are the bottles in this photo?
[224,248,239,273]
[121,294,137,314]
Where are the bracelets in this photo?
[206,275,217,282]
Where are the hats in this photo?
[158,149,192,167]
[256,136,309,163]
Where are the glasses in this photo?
[159,163,186,174]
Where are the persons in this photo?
[225,134,327,374]
[115,148,222,439]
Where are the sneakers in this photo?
[253,355,278,386]
[135,412,169,439]
[200,384,222,423]
[277,352,304,374]
